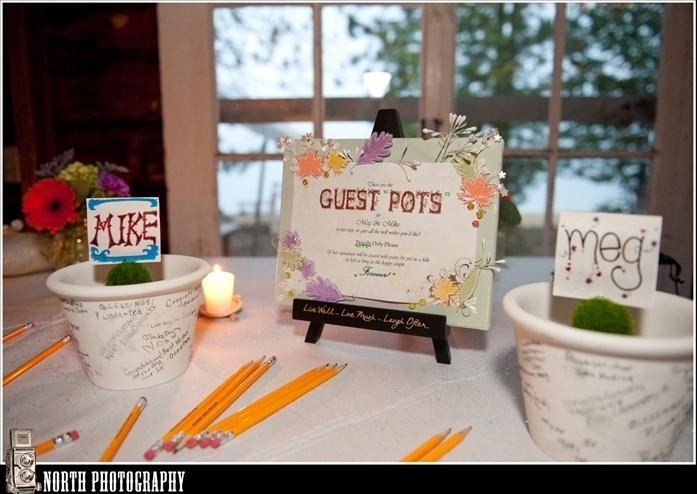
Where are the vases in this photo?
[42,229,89,271]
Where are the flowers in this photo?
[21,151,131,236]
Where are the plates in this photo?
[198,296,247,319]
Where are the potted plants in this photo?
[500,279,695,463]
[43,251,208,389]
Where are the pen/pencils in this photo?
[3,322,71,387]
[401,426,473,463]
[99,396,146,461]
[143,355,347,460]
[36,430,78,455]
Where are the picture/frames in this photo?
[271,136,505,332]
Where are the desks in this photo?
[4,252,697,463]
